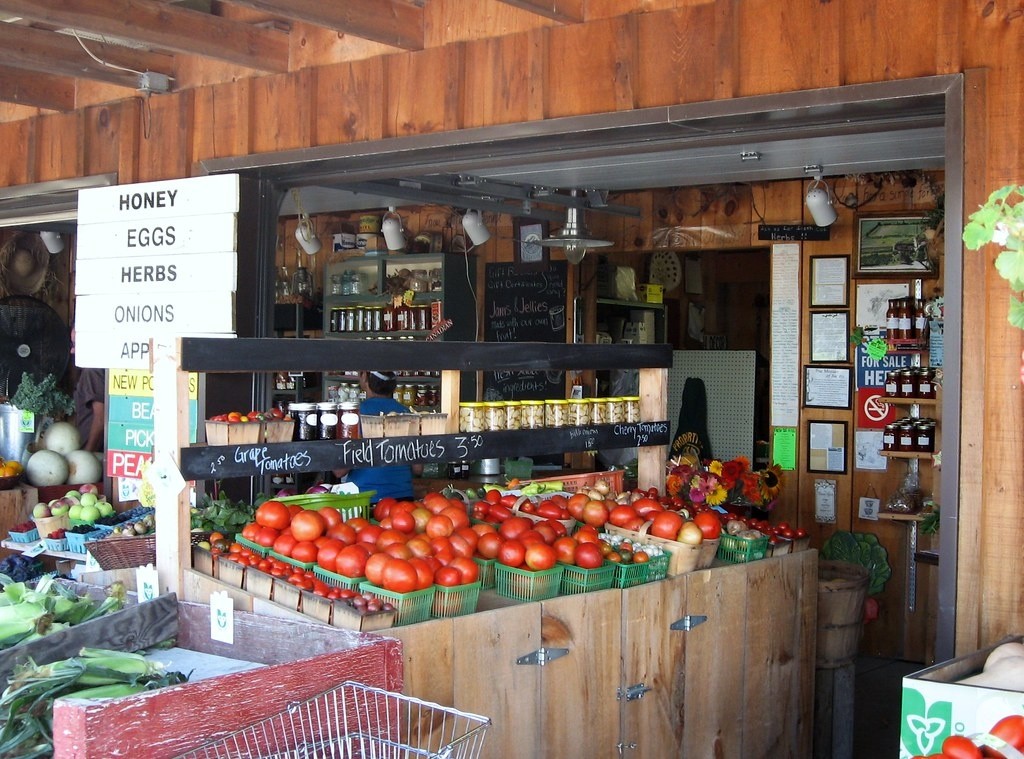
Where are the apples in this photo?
[33,484,112,521]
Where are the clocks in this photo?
[512,215,551,274]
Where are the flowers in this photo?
[667,458,784,512]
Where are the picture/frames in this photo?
[850,210,940,280]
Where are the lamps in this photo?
[295,213,322,256]
[532,190,615,265]
[380,206,406,250]
[39,230,65,254]
[805,174,839,228]
[463,208,491,246]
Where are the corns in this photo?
[0,597,153,699]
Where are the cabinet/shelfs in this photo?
[272,303,323,403]
[180,549,819,758]
[321,253,477,415]
[178,339,672,481]
[877,339,936,526]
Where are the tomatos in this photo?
[209,409,293,423]
[242,488,806,621]
[911,715,1024,759]
[0,457,23,477]
[199,531,397,613]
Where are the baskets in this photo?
[235,470,769,626]
[8,504,230,572]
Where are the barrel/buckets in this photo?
[814,560,871,668]
[0,402,47,480]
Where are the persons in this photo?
[332,371,423,508]
[70,327,106,452]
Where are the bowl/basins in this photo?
[1,475,23,490]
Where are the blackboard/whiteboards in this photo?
[181,420,671,482]
[758,224,830,241]
[181,337,673,372]
[482,260,568,403]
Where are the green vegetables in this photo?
[11,371,76,416]
[191,490,272,542]
[822,513,940,595]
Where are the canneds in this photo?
[289,401,360,441]
[459,396,641,433]
[330,307,439,407]
[884,366,936,398]
[882,416,936,453]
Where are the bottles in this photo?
[275,250,313,300]
[331,271,364,294]
[328,304,439,407]
[886,297,929,339]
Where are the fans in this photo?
[0,294,70,398]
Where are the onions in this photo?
[113,514,155,536]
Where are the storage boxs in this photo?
[636,284,663,304]
[193,421,810,632]
[8,517,134,554]
